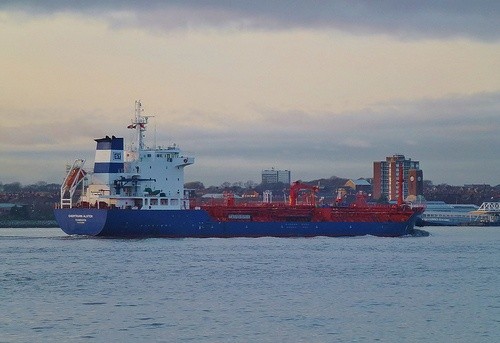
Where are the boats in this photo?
[53,96,427,237]
